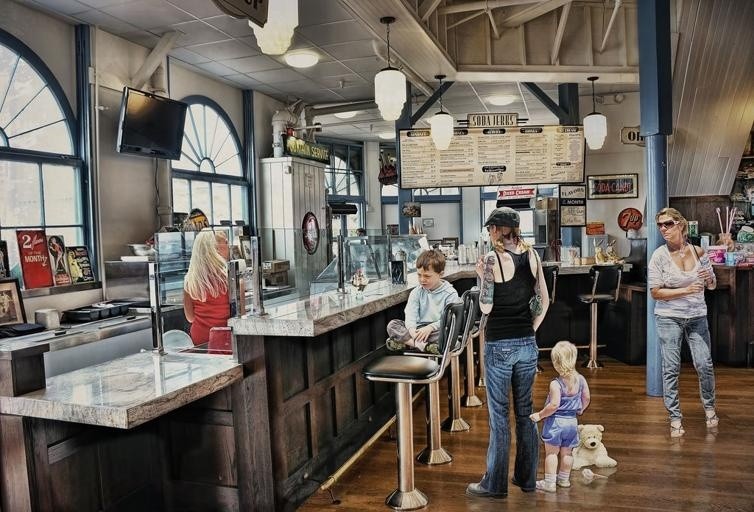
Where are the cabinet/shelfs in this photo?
[259,157,329,294]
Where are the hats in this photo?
[484,207,520,228]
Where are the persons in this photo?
[181,226,232,345]
[529,339,592,493]
[384,250,459,354]
[647,208,720,438]
[464,207,550,502]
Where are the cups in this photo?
[723,252,735,266]
[457,244,477,264]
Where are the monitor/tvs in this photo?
[116,86,188,161]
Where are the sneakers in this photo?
[426,343,440,354]
[386,337,407,352]
[556,474,571,488]
[536,480,556,493]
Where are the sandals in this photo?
[670,423,684,437]
[705,414,719,425]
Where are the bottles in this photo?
[396,249,407,261]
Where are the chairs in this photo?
[359,286,488,511]
[575,264,624,369]
[531,263,560,374]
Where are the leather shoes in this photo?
[512,478,534,492]
[466,483,506,498]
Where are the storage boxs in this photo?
[261,258,291,274]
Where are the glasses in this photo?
[656,220,680,228]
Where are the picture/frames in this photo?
[587,174,638,200]
[442,237,458,260]
[212,224,252,268]
[0,276,27,328]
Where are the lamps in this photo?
[581,76,608,151]
[247,1,298,56]
[372,16,407,122]
[430,75,454,151]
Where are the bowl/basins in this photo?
[125,242,177,257]
